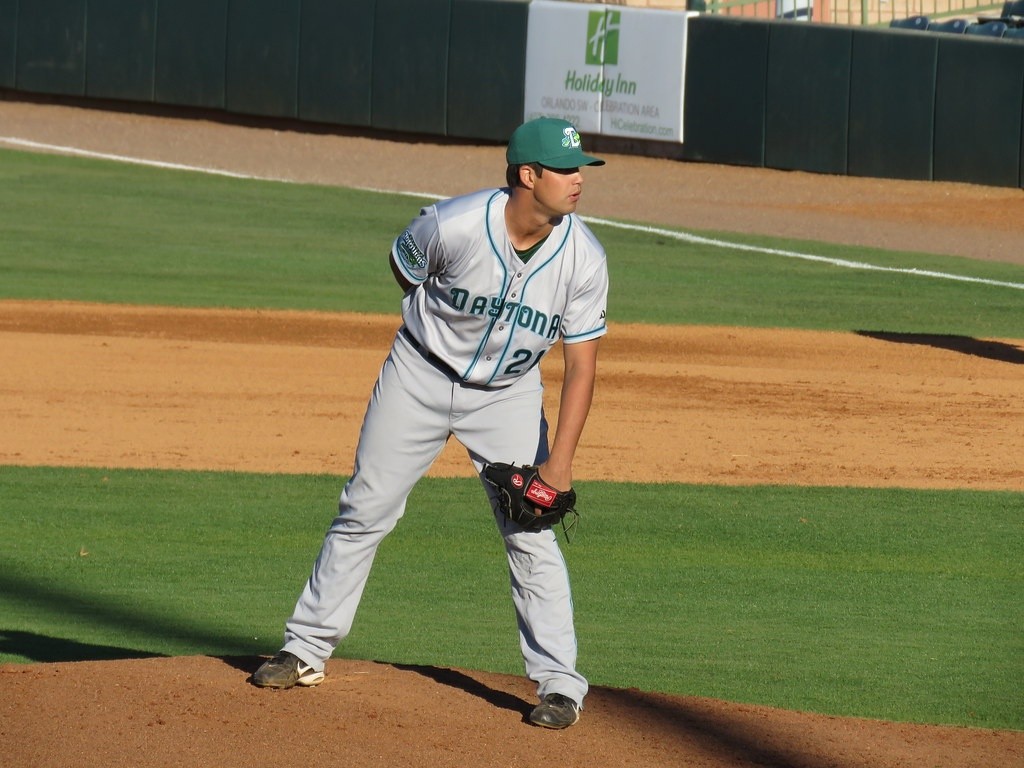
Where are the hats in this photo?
[505,115,605,170]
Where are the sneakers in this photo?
[254,650,324,689]
[530,692,581,729]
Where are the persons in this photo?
[251,116,609,727]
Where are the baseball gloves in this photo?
[481,460,580,545]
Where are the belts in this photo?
[402,326,458,377]
